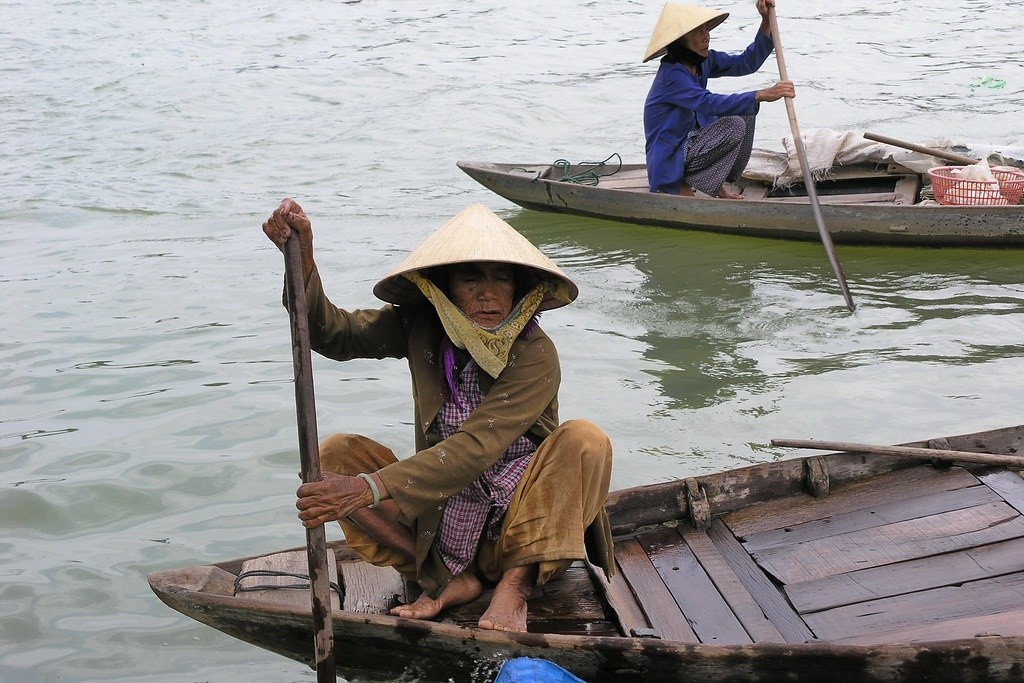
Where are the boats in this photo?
[149,422,1024,683]
[459,143,1024,248]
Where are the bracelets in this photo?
[356,472,379,508]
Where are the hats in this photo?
[643,2,729,65]
[373,203,580,312]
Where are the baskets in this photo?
[928,165,1024,205]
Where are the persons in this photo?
[642,0,796,198]
[261,198,614,635]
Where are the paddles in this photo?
[766,6,857,313]
[282,231,339,683]
